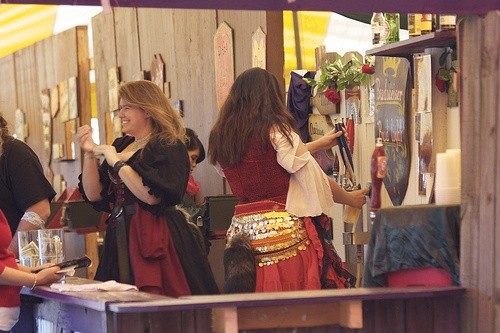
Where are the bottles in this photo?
[407,13,423,39]
[421,14,432,34]
[384,12,400,44]
[371,12,386,48]
[367,138,386,212]
[431,13,439,32]
[439,13,457,30]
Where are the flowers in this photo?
[433,39,458,94]
[302,53,376,105]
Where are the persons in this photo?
[207,67,370,293]
[0,115,59,262]
[0,207,65,333]
[184,127,207,224]
[76,79,218,297]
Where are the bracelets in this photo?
[30,273,37,291]
[113,159,128,173]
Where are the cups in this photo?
[18,229,40,267]
[435,148,461,205]
[37,227,66,285]
[343,204,361,224]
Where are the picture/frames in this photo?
[103,109,125,146]
[62,118,77,161]
